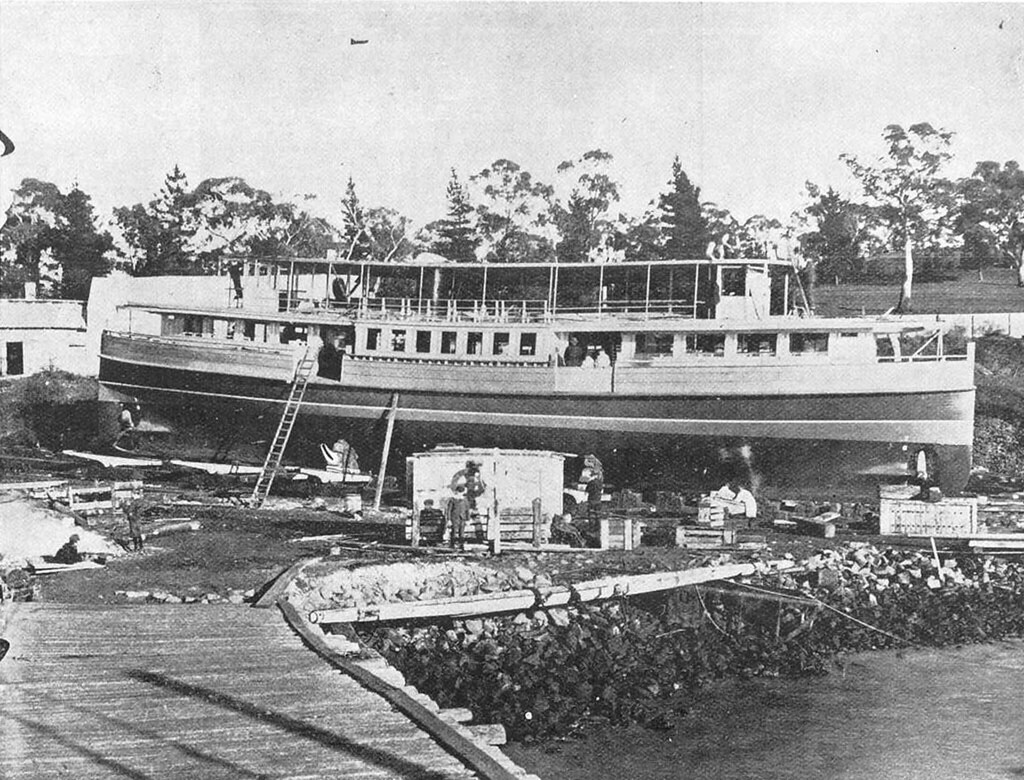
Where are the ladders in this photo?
[246,345,323,513]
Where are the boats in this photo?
[96,253,977,501]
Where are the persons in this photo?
[584,471,603,532]
[446,486,469,550]
[52,534,89,564]
[229,260,243,299]
